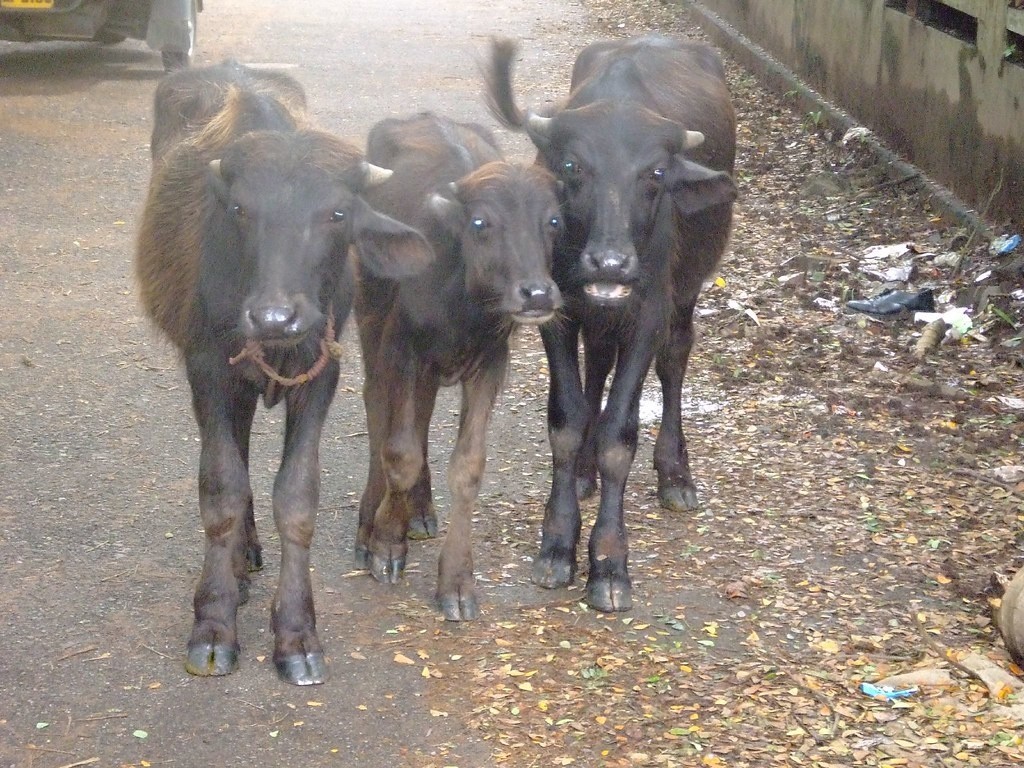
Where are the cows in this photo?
[476,33,740,610]
[354,112,564,623]
[135,61,393,682]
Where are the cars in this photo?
[0,0,202,75]
